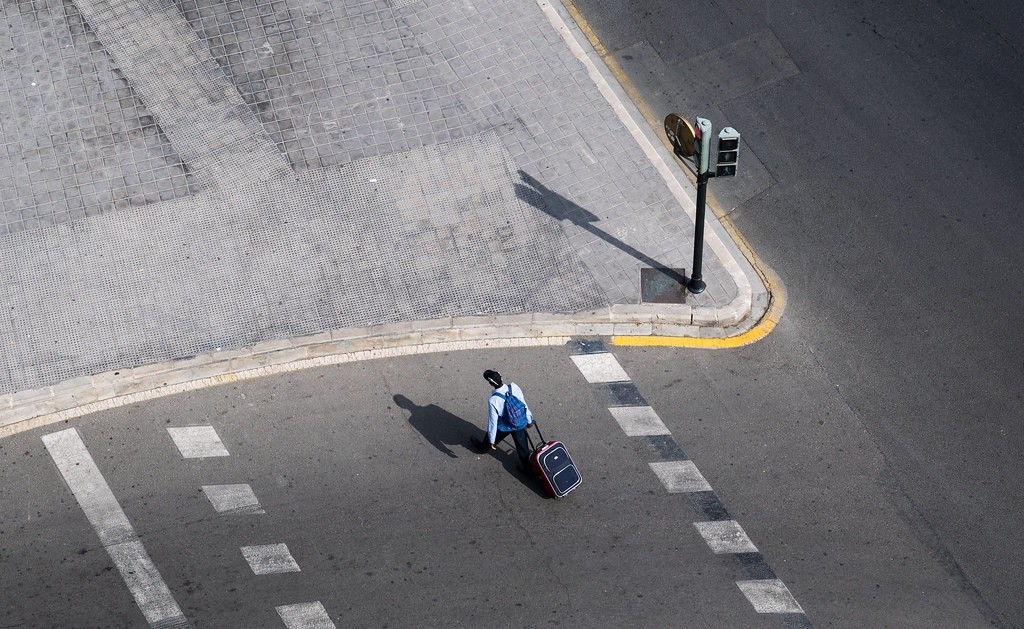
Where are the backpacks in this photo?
[493,384,528,431]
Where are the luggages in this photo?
[523,420,583,499]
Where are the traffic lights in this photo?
[717,136,738,178]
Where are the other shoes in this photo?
[516,464,530,476]
[470,435,488,453]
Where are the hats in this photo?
[483,370,502,386]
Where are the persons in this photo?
[471,369,537,479]
